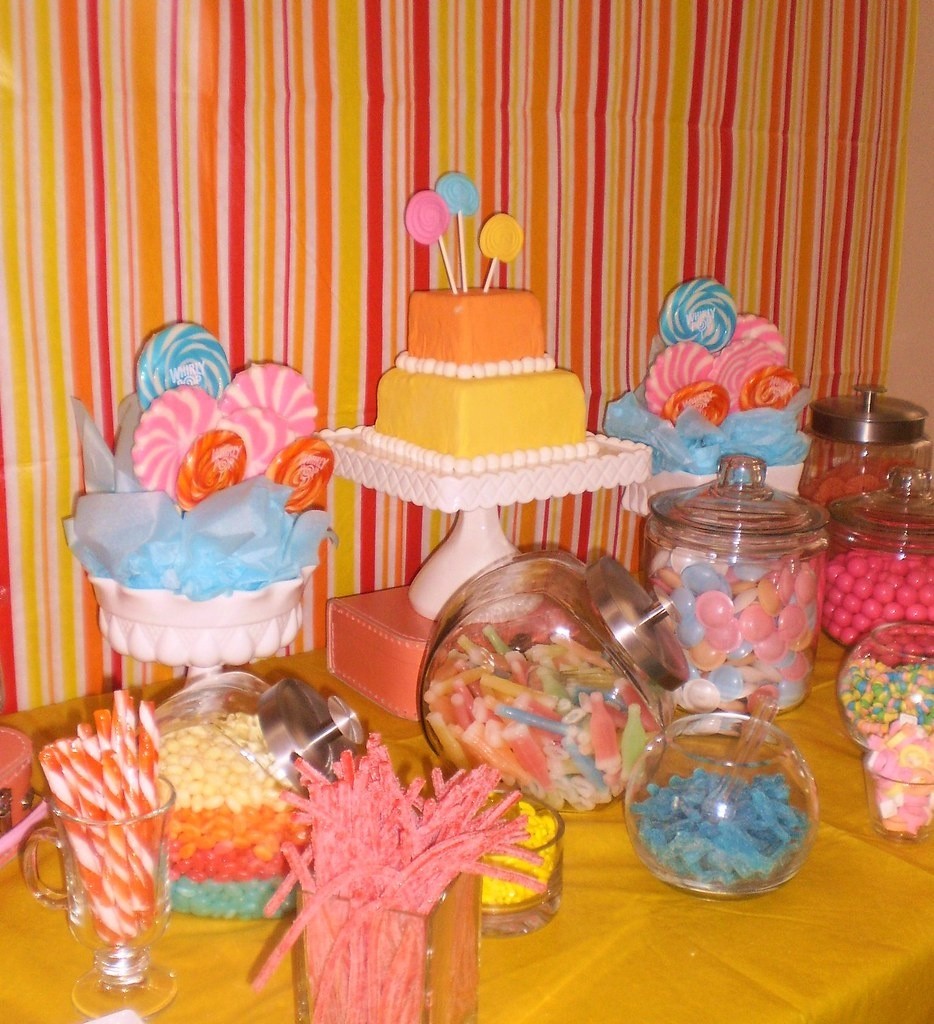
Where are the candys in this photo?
[404,170,523,263]
[35,456,934,1024]
[131,321,335,512]
[644,279,801,428]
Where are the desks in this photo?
[1,617,934,1023]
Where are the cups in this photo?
[863,753,934,844]
[25,775,177,1019]
[294,865,482,1024]
[477,786,566,934]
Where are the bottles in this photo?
[797,382,932,516]
[643,454,830,716]
[836,622,933,749]
[416,553,691,814]
[625,711,821,897]
[154,669,365,922]
[819,465,934,649]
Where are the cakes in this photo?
[364,287,599,472]
